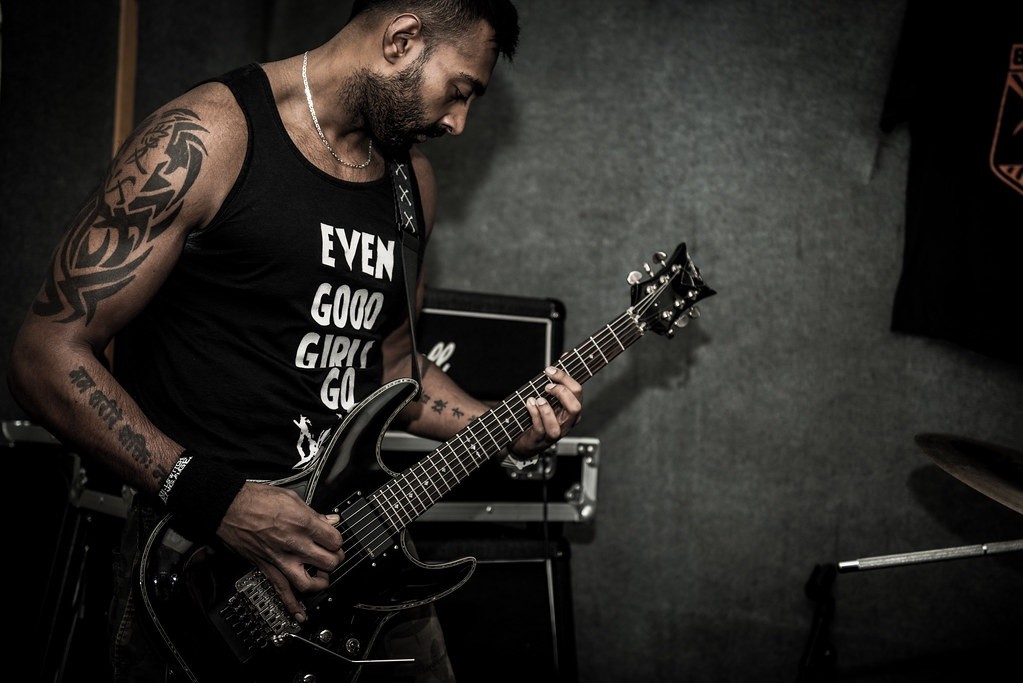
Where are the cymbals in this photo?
[914,433,1023,517]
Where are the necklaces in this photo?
[302,50,372,169]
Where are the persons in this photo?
[12,0,584,683]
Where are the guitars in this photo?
[135,241,722,683]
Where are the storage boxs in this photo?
[378,431,601,524]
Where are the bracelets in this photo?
[152,448,248,546]
